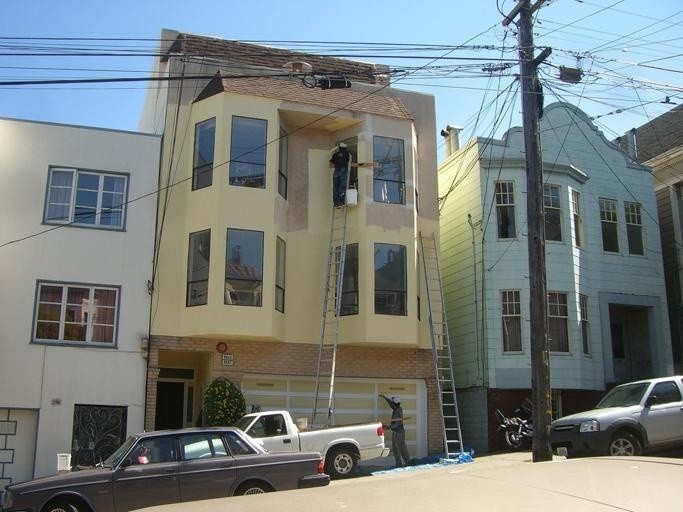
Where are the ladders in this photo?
[311,152,353,429]
[419,231,465,460]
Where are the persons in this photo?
[328,140,351,210]
[378,393,410,466]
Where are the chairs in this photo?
[271,420,280,434]
[159,442,172,461]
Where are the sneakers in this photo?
[394,460,411,467]
[333,204,345,210]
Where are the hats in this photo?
[391,396,401,404]
[339,142,348,148]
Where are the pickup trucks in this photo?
[184,410,390,478]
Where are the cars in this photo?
[1,427,331,512]
[549,375,683,458]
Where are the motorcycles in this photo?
[495,408,534,452]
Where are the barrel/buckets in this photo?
[58,454,72,472]
[296,418,307,430]
[346,187,357,207]
